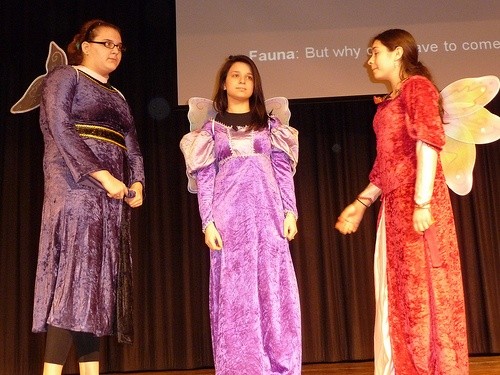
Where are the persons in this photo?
[30,19,146,375]
[334,28,472,375]
[178,54,306,374]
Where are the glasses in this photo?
[88,40,126,51]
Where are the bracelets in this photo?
[355,198,370,209]
[413,199,433,209]
